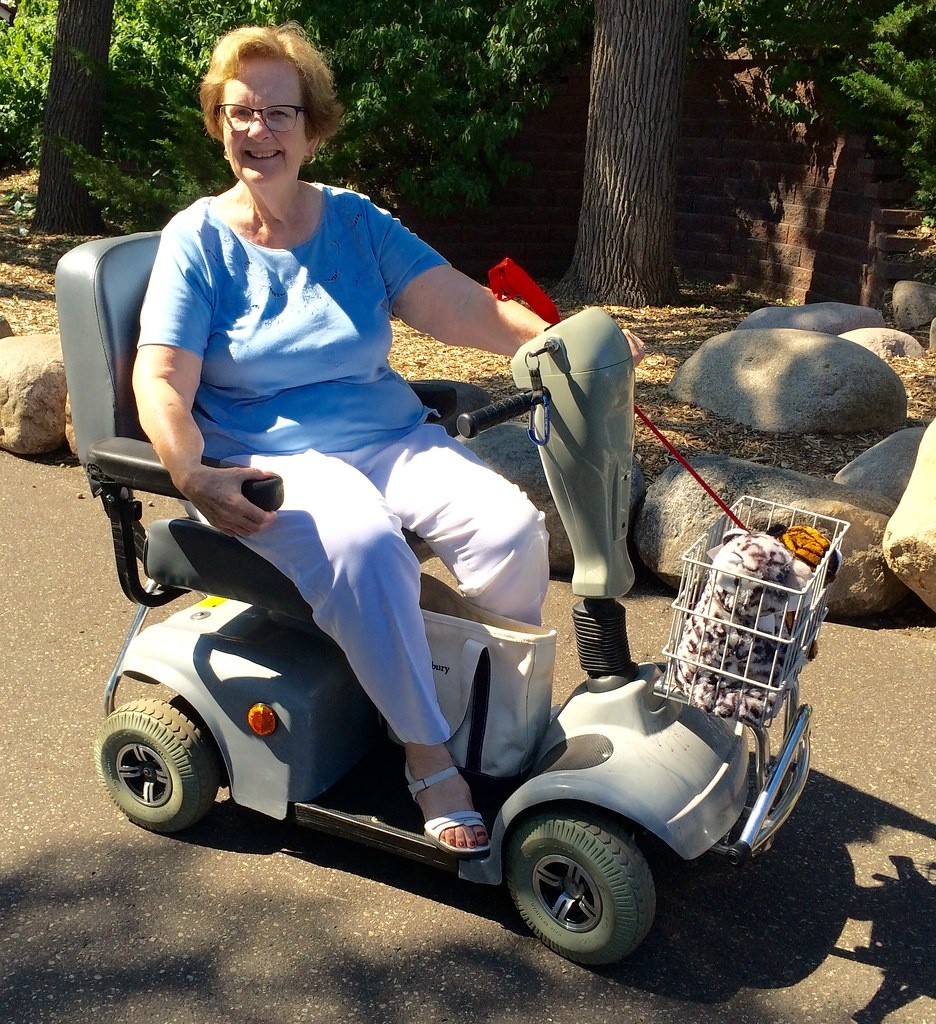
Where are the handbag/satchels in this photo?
[377,571,556,777]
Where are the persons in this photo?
[128,24,649,861]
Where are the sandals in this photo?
[400,752,495,858]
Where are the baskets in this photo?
[647,494,855,728]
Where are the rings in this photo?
[637,347,644,351]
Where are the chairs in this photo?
[55,230,488,626]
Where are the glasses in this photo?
[210,99,308,135]
